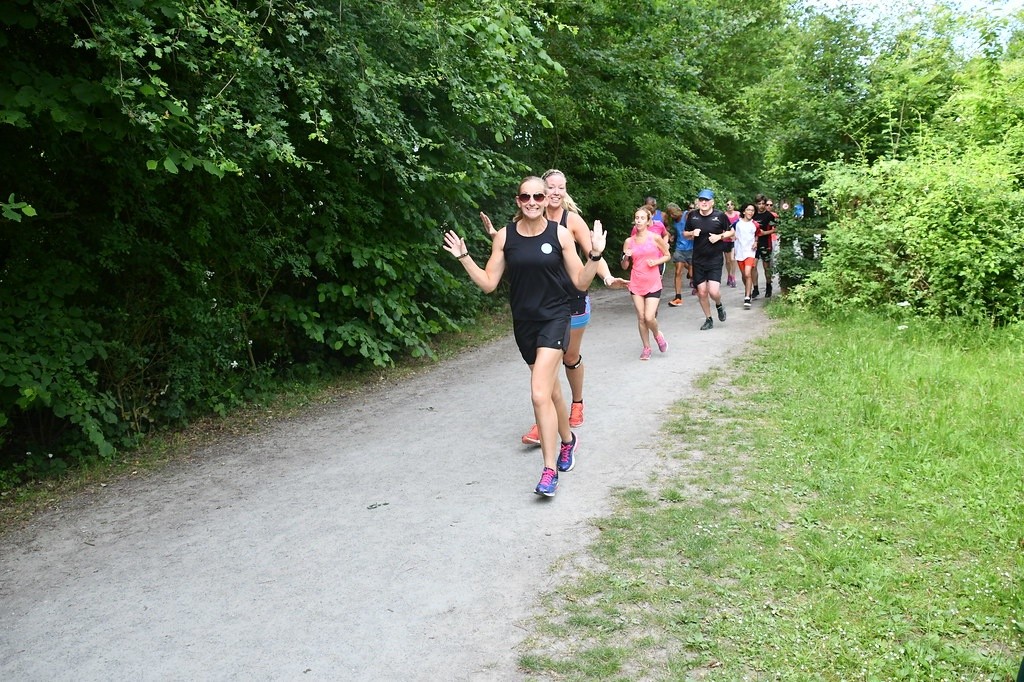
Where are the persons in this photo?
[443,176,607,497]
[480,169,630,446]
[620,189,804,359]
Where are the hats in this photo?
[698,190,714,200]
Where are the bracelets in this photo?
[720,234,724,239]
[589,251,602,261]
[456,253,469,260]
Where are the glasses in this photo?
[726,204,733,206]
[519,193,546,202]
[766,204,773,207]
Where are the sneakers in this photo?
[654,331,668,353]
[534,465,559,496]
[569,399,584,428]
[700,320,714,330]
[716,303,726,321]
[668,299,683,306]
[557,430,577,472]
[640,347,652,360]
[522,425,540,446]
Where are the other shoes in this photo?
[744,298,751,307]
[764,286,772,299]
[727,277,736,286]
[686,272,689,278]
[689,282,693,288]
[692,289,697,295]
[749,290,760,298]
